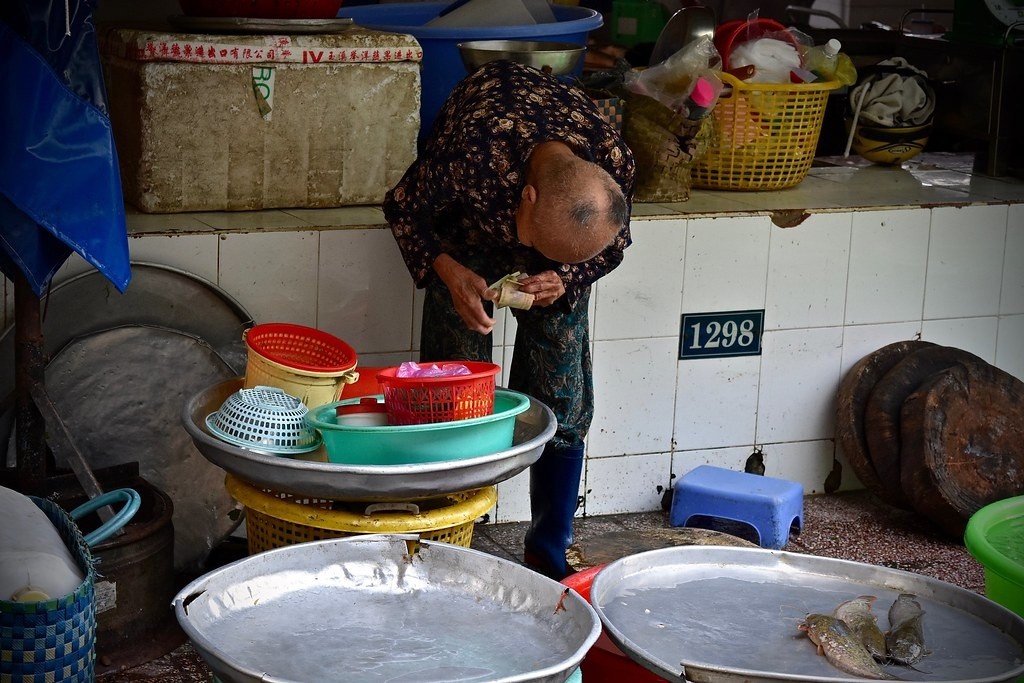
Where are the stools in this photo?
[668,464,805,548]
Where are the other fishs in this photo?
[798,593,933,681]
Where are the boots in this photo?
[525,441,585,585]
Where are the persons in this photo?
[381,60,635,582]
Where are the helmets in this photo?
[850,113,933,167]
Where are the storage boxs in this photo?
[104,28,423,212]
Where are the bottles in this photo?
[802,38,841,84]
[0,486,86,602]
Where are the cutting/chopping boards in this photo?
[837,342,1024,533]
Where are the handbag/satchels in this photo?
[604,77,714,202]
[586,88,625,137]
[0,488,141,683]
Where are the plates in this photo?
[171,533,603,683]
[589,545,1024,683]
[167,12,352,33]
[179,378,565,502]
[0,261,256,570]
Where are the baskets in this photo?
[205,385,324,453]
[375,361,500,425]
[224,472,497,556]
[691,20,842,191]
[247,323,357,372]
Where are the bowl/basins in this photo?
[843,116,934,165]
[304,390,531,465]
[337,0,604,143]
[964,494,1024,621]
[456,39,587,75]
[559,562,671,683]
[648,5,717,66]
[179,0,342,20]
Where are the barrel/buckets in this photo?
[242,327,359,413]
